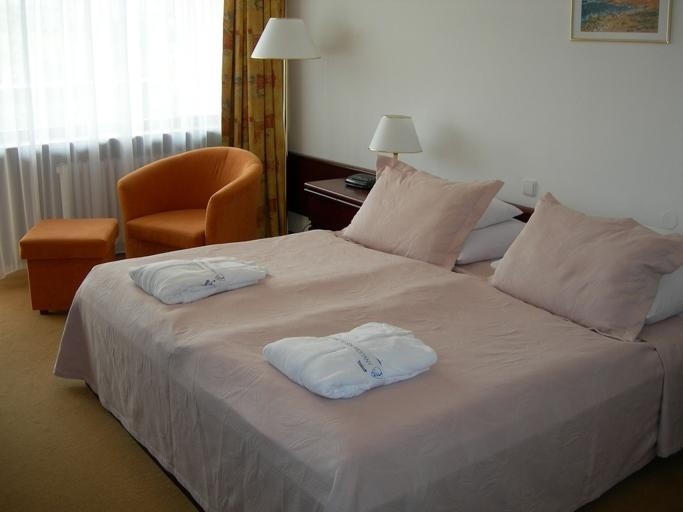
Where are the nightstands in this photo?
[302,177,369,231]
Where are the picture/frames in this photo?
[569,0,673,45]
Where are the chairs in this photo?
[117,145,263,259]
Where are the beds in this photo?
[51,229,683,512]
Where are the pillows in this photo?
[471,196,523,229]
[644,265,682,326]
[455,217,525,266]
[489,191,683,343]
[335,158,505,271]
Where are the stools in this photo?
[18,217,120,315]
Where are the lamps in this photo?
[368,114,424,162]
[250,16,322,232]
